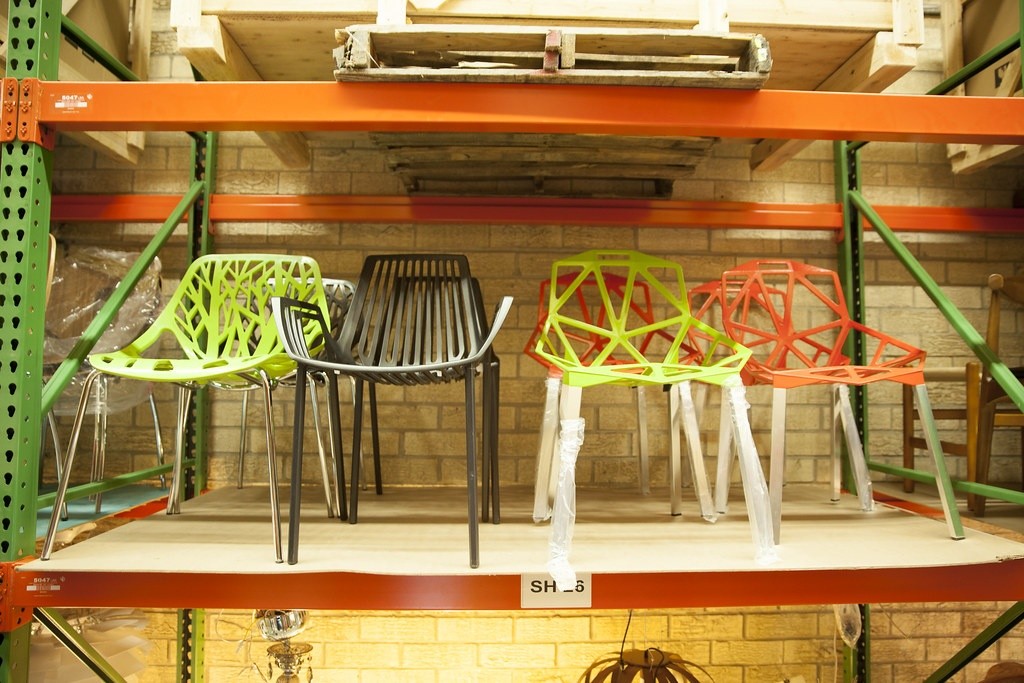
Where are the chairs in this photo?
[36,248,969,589]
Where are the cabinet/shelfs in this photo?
[0,0,1024,683]
